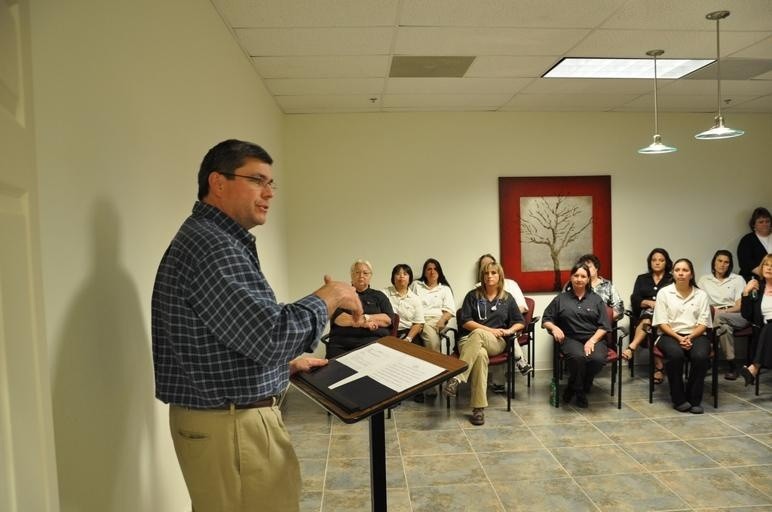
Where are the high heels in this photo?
[740,366,755,387]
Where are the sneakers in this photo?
[492,380,506,393]
[562,377,589,407]
[444,377,458,398]
[725,367,738,381]
[470,407,485,425]
[424,387,439,398]
[689,406,705,414]
[413,393,423,404]
[515,357,534,376]
[673,401,692,412]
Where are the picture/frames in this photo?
[499,177,611,292]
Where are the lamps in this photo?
[638,49,677,154]
[695,10,744,140]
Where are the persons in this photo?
[330,258,454,397]
[541,248,772,414]
[736,207,771,288]
[470,254,533,392]
[150,139,366,510]
[442,262,528,425]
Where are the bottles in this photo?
[750,276,759,301]
[549,377,559,406]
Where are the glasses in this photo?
[218,171,279,191]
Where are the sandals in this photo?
[654,367,665,384]
[622,345,635,361]
